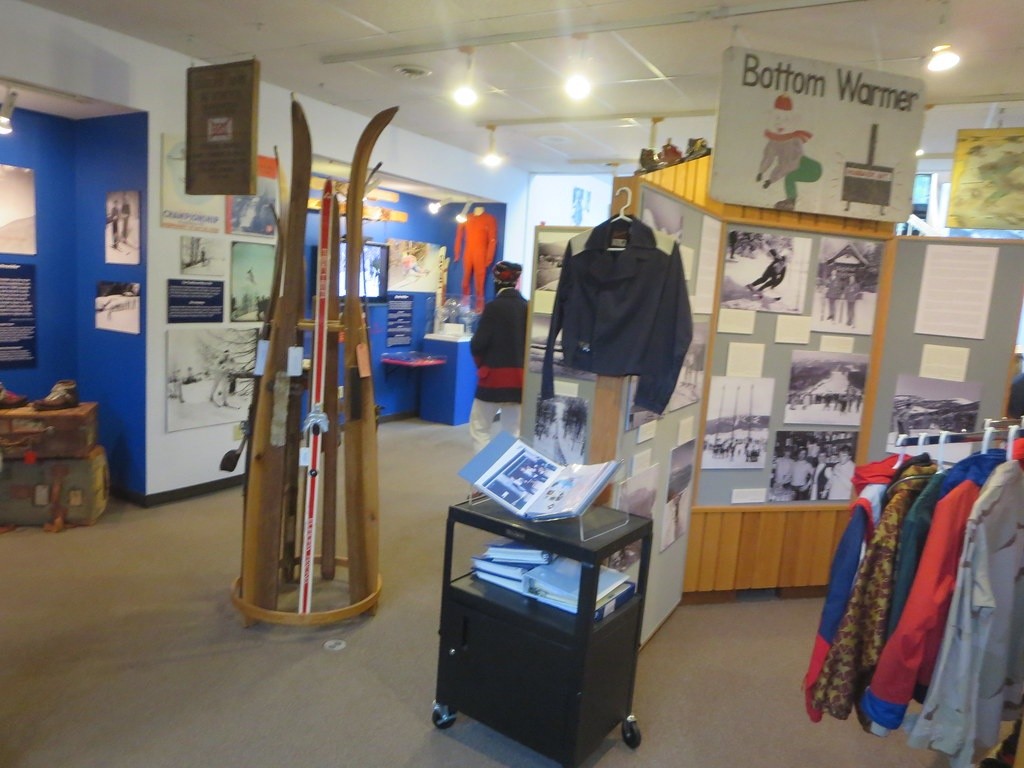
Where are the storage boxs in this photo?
[0,399,111,532]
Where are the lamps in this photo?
[927,44,960,73]
[428,197,453,212]
[455,200,473,222]
[452,58,479,105]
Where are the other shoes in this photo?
[747,285,754,290]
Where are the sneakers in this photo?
[662,144,682,165]
[34,379,77,410]
[0,383,30,409]
[640,148,658,171]
[684,138,707,162]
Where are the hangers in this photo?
[887,418,1024,482]
[561,185,681,260]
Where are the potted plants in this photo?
[443,298,465,338]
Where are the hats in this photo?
[492,261,522,283]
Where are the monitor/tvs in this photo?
[338,238,389,303]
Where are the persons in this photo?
[111,199,130,248]
[745,249,785,294]
[404,252,430,275]
[713,438,761,462]
[825,270,860,325]
[455,207,496,313]
[772,447,854,500]
[727,230,736,259]
[510,466,548,494]
[200,248,204,263]
[469,261,528,452]
[210,350,235,406]
[789,390,863,412]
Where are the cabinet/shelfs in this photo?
[431,493,653,768]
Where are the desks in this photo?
[382,350,447,384]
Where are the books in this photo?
[472,536,635,621]
[458,431,623,520]
